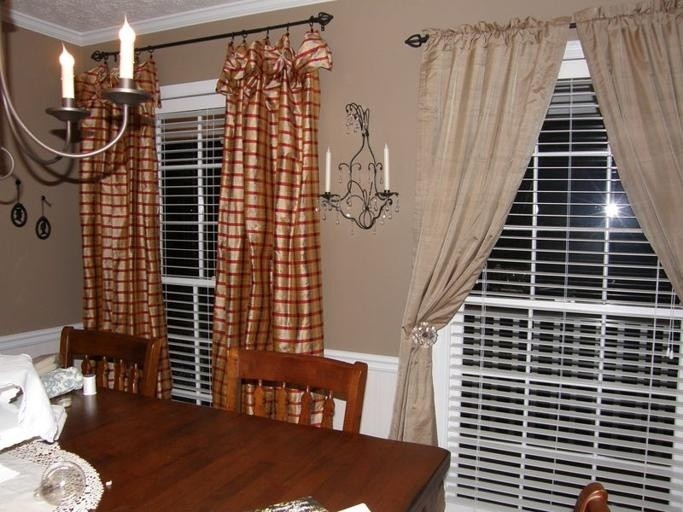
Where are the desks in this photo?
[0,386,450,512]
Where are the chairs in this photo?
[59,326,161,399]
[225,348,368,434]
[574,482,611,512]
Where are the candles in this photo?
[384,142,390,191]
[325,147,331,192]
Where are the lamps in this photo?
[0,0,148,180]
